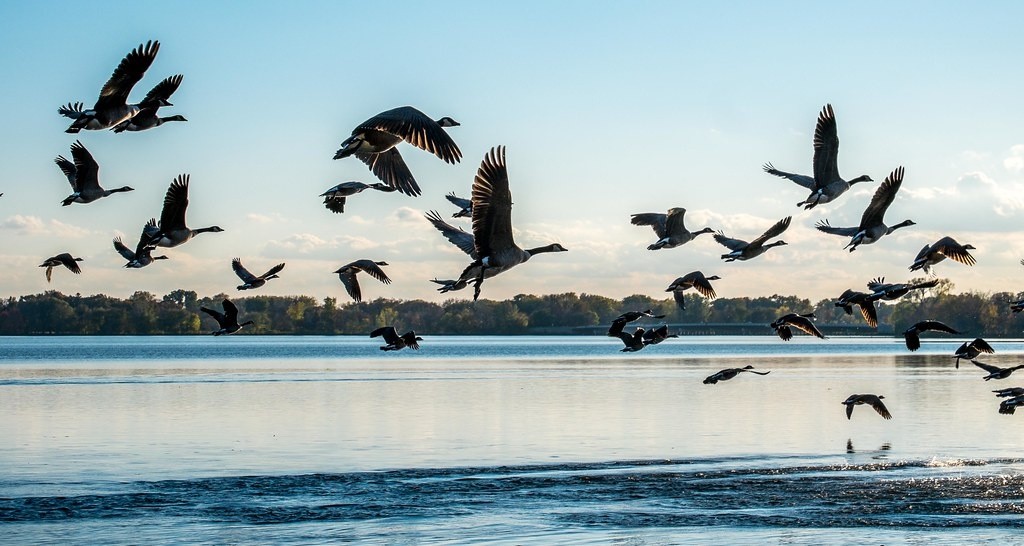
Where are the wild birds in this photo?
[53,139,135,207]
[703,365,770,385]
[332,105,463,197]
[606,309,679,353]
[112,217,170,269]
[423,144,568,301]
[231,256,286,291]
[332,258,393,302]
[369,325,424,351]
[444,190,514,219]
[713,215,792,263]
[629,208,715,250]
[56,39,188,135]
[991,387,1024,415]
[761,103,1024,422]
[147,173,225,248]
[38,252,85,284]
[317,182,399,214]
[199,297,255,337]
[664,270,721,310]
